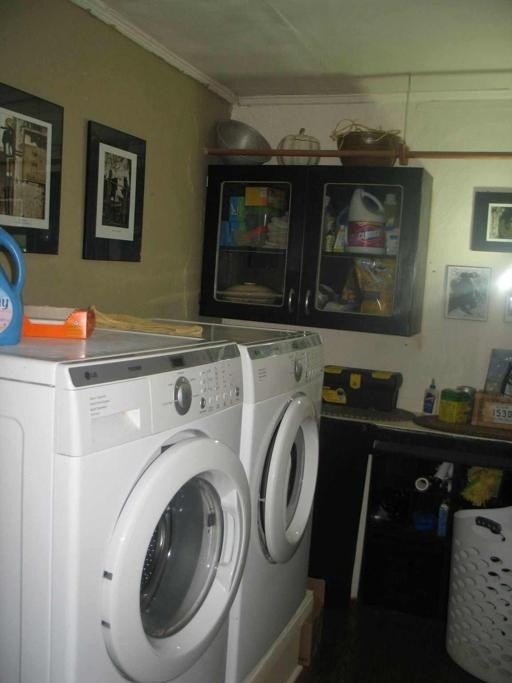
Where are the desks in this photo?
[320,397,511,614]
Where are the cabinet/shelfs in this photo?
[197,164,435,338]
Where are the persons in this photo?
[102,159,129,228]
[493,206,512,239]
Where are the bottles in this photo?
[437,502,449,536]
[382,192,398,226]
[421,378,440,415]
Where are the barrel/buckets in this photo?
[347,188,384,255]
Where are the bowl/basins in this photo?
[220,283,275,305]
[214,117,273,165]
[336,132,401,167]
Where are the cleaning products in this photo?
[1,228,28,347]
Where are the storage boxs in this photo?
[298,576,327,670]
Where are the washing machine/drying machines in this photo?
[87,314,325,683]
[0,317,254,683]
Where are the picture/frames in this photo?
[470,190,512,252]
[80,119,147,263]
[0,83,63,254]
[446,265,493,321]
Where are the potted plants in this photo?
[330,118,404,166]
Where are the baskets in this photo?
[445,506,512,682]
